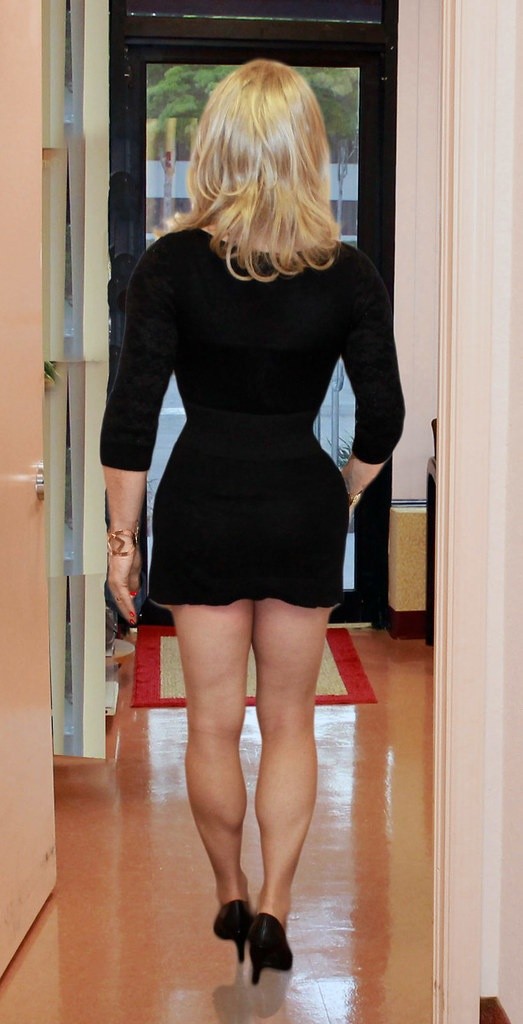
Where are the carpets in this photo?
[130,623,377,707]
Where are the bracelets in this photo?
[106,520,139,557]
[341,470,362,512]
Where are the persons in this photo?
[100,60,405,986]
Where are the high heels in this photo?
[247,912,293,984]
[213,900,252,962]
